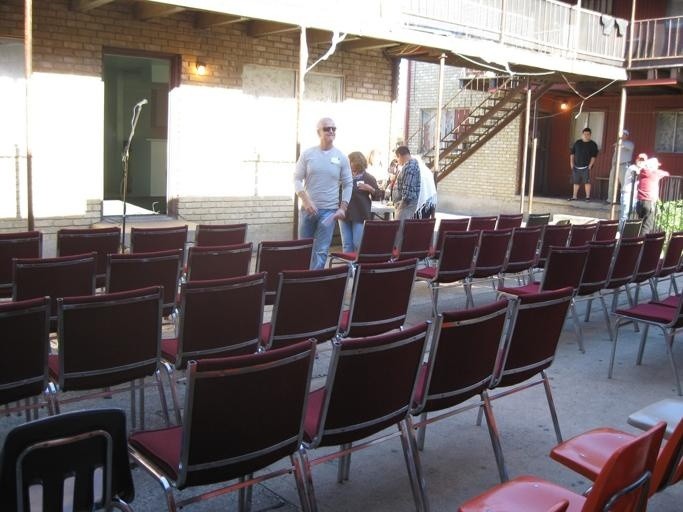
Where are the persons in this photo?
[410,154,438,262]
[614,152,647,240]
[386,146,422,263]
[636,157,670,238]
[336,150,385,262]
[567,128,599,202]
[604,130,635,205]
[292,115,353,271]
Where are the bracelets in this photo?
[340,201,349,211]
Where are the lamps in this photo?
[197,61,207,75]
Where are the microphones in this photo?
[137,99,148,106]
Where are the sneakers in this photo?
[568,197,578,200]
[586,197,590,202]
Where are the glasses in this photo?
[322,127,336,131]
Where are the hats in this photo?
[636,153,648,162]
[624,129,629,135]
[646,158,661,165]
